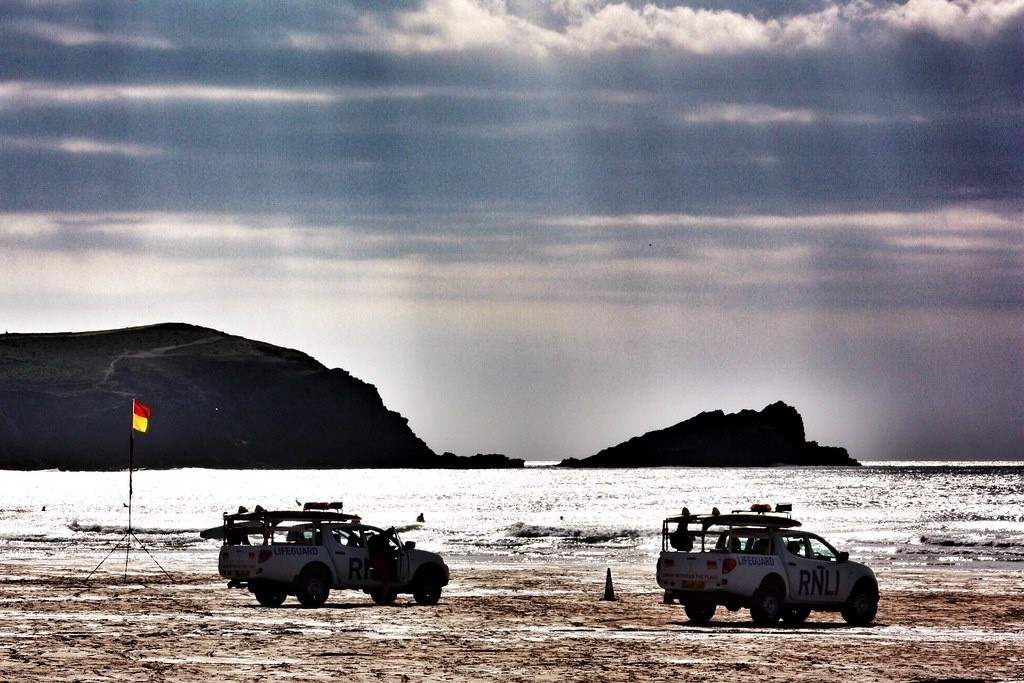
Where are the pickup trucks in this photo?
[656,504,880,618]
[199,501,450,609]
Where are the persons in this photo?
[369,528,398,578]
[788,541,802,557]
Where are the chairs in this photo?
[286,531,304,544]
[677,540,693,553]
[753,541,774,554]
[315,532,323,545]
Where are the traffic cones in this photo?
[597,568,621,595]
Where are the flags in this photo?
[133,402,150,432]
